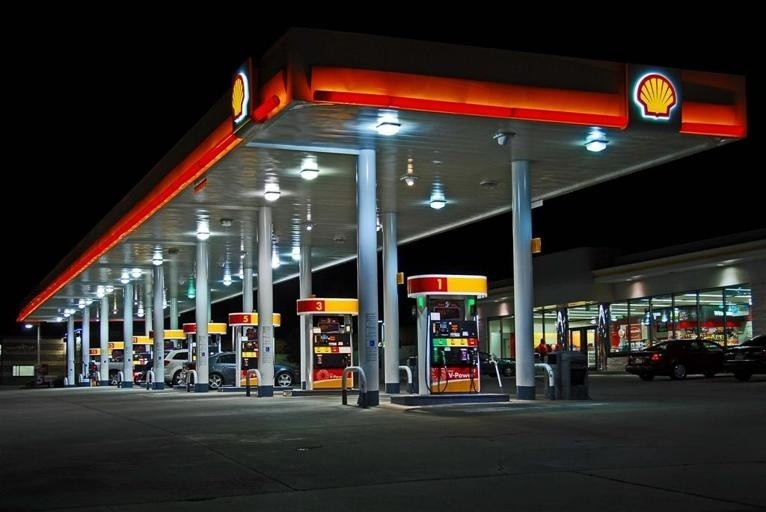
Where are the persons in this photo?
[538,339,548,357]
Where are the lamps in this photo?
[197,232,210,241]
[375,121,399,135]
[152,259,163,266]
[298,169,319,181]
[430,200,445,209]
[264,190,280,201]
[586,140,609,153]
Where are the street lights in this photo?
[24,322,41,365]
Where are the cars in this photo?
[92,347,295,390]
[724,335,766,378]
[409,348,516,377]
[625,340,729,381]
